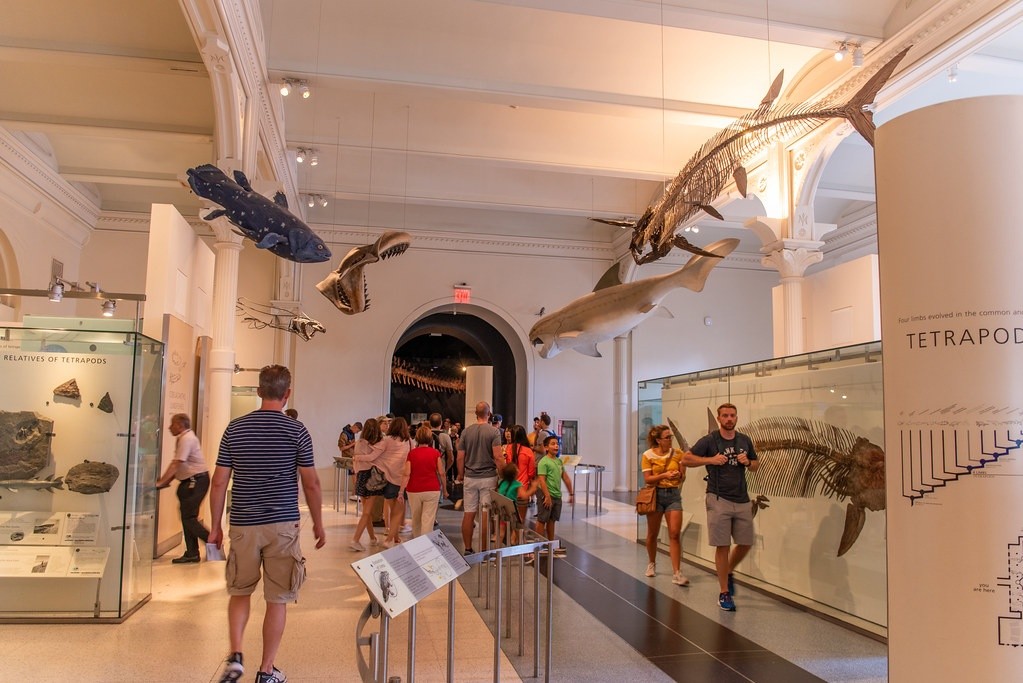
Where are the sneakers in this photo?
[728,572,735,596]
[645,562,656,578]
[672,569,690,586]
[717,592,736,612]
[217,653,244,683]
[253,666,287,683]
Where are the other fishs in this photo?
[0,472,65,495]
[185,162,333,264]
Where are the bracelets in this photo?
[679,472,683,479]
[745,459,751,467]
[398,491,404,495]
[570,494,574,496]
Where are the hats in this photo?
[493,414,503,422]
[533,416,540,421]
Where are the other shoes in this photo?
[523,554,535,566]
[539,542,550,554]
[347,541,367,552]
[482,551,496,563]
[383,537,402,548]
[348,494,362,502]
[371,537,378,546]
[554,545,566,553]
[464,548,475,556]
[172,551,201,564]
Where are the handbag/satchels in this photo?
[366,465,388,491]
[635,487,657,516]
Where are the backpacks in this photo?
[541,430,562,459]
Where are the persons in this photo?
[682,402,760,611]
[534,435,574,542]
[206,366,327,683]
[284,409,298,420]
[641,425,690,586]
[638,417,656,455]
[337,400,556,564]
[143,413,211,563]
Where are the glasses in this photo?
[658,434,675,441]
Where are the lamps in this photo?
[296,146,320,166]
[85,281,117,317]
[307,193,328,208]
[234,364,240,375]
[280,78,311,100]
[834,40,863,68]
[48,276,84,302]
